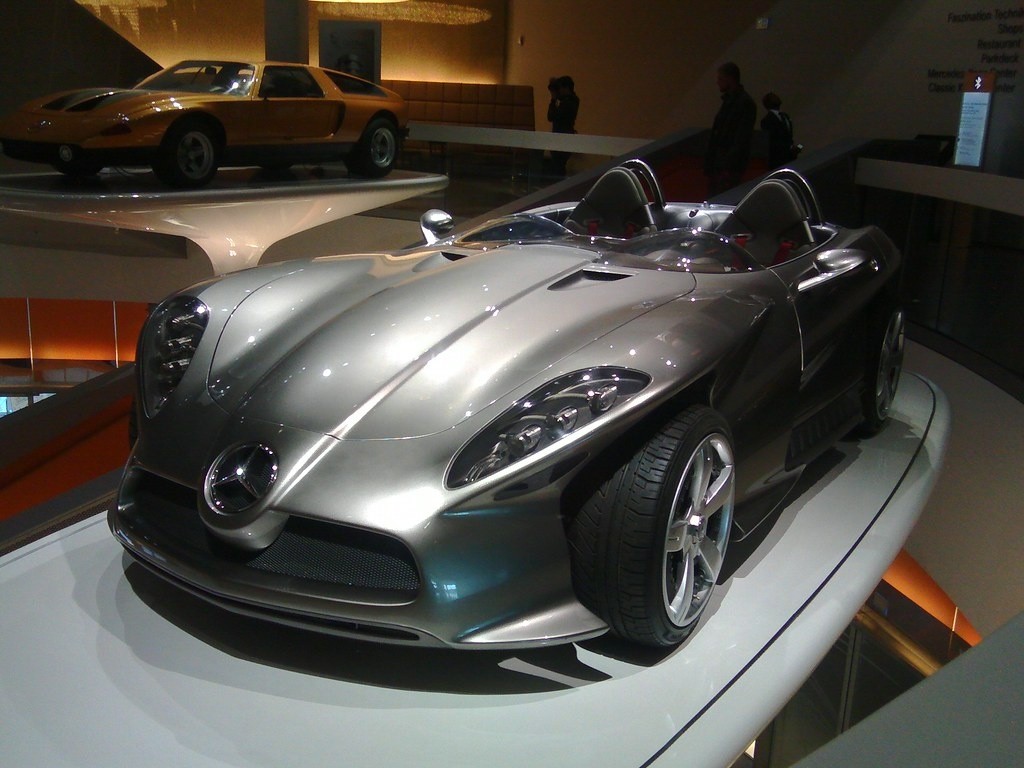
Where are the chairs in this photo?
[562,159,664,242]
[715,168,820,267]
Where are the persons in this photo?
[547,75,579,180]
[702,62,757,196]
[761,91,798,165]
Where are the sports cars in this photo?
[0,57,413,193]
[106,154,913,657]
